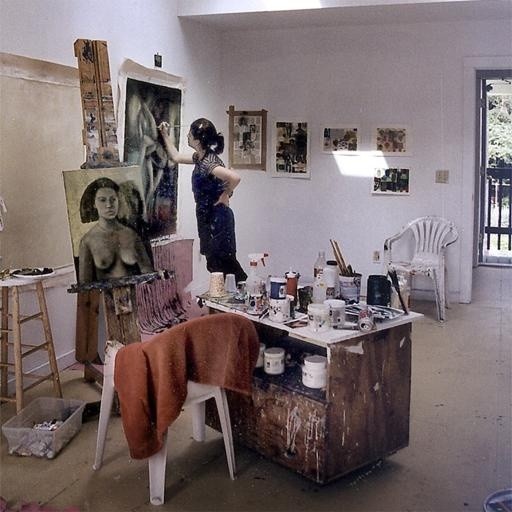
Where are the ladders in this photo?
[0,282,63,429]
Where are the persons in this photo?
[127,85,181,207]
[77,176,155,284]
[234,116,409,194]
[157,118,249,287]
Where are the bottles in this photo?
[366,250,389,306]
[312,250,339,303]
[285,266,298,303]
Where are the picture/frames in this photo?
[370,165,411,195]
[320,123,361,155]
[371,125,410,156]
[270,117,312,179]
[225,104,267,171]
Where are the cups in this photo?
[208,272,225,299]
[224,274,237,294]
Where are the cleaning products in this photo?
[245,253,268,315]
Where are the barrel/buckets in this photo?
[325,299,345,326]
[367,274,389,305]
[308,303,328,331]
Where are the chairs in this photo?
[382,215,459,322]
[92,305,257,505]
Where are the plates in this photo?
[11,267,55,279]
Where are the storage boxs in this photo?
[5,398,87,459]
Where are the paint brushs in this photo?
[155,124,187,127]
[329,239,355,276]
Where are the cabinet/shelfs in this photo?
[202,289,422,484]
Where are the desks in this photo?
[1,275,61,415]
